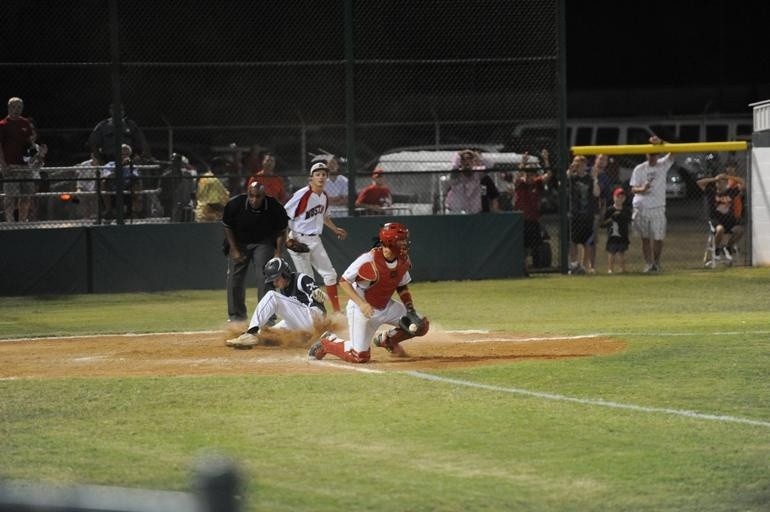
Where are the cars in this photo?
[512,114,754,202]
[2,151,197,222]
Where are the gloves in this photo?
[311,290,327,304]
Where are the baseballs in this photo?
[409,323,418,332]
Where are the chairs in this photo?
[703,221,740,269]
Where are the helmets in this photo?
[379,222,409,255]
[264,257,293,283]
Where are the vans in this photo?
[367,146,548,214]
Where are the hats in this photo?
[614,186,625,196]
[371,169,386,179]
[310,162,329,176]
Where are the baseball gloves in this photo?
[399,310,424,337]
[286,240,310,253]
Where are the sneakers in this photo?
[309,331,333,359]
[577,260,668,275]
[226,333,260,349]
[713,245,734,261]
[374,333,406,357]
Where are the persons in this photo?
[724,161,749,252]
[220,256,330,349]
[157,150,230,223]
[0,96,43,225]
[24,126,47,221]
[242,143,287,207]
[696,172,747,262]
[75,144,144,219]
[564,154,601,275]
[276,163,350,320]
[321,157,348,218]
[354,170,393,216]
[510,146,555,273]
[82,103,152,227]
[597,187,635,275]
[219,183,287,327]
[565,151,612,273]
[623,135,683,272]
[306,220,429,362]
[443,149,502,214]
